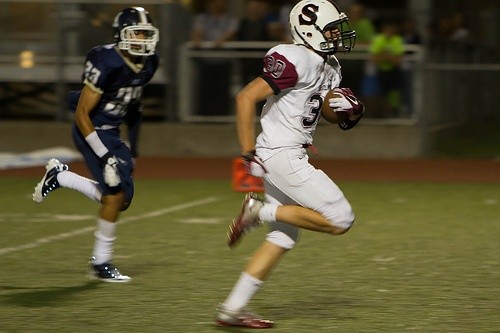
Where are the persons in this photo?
[31,6,160,285]
[212,0,367,328]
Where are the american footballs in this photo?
[321,89,348,123]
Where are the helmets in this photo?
[289,0,348,56]
[112,6,153,43]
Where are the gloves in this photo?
[328,87,363,115]
[99,151,128,189]
[242,149,266,177]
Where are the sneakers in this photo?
[226,191,264,249]
[32,158,68,203]
[86,262,130,283]
[216,304,274,329]
